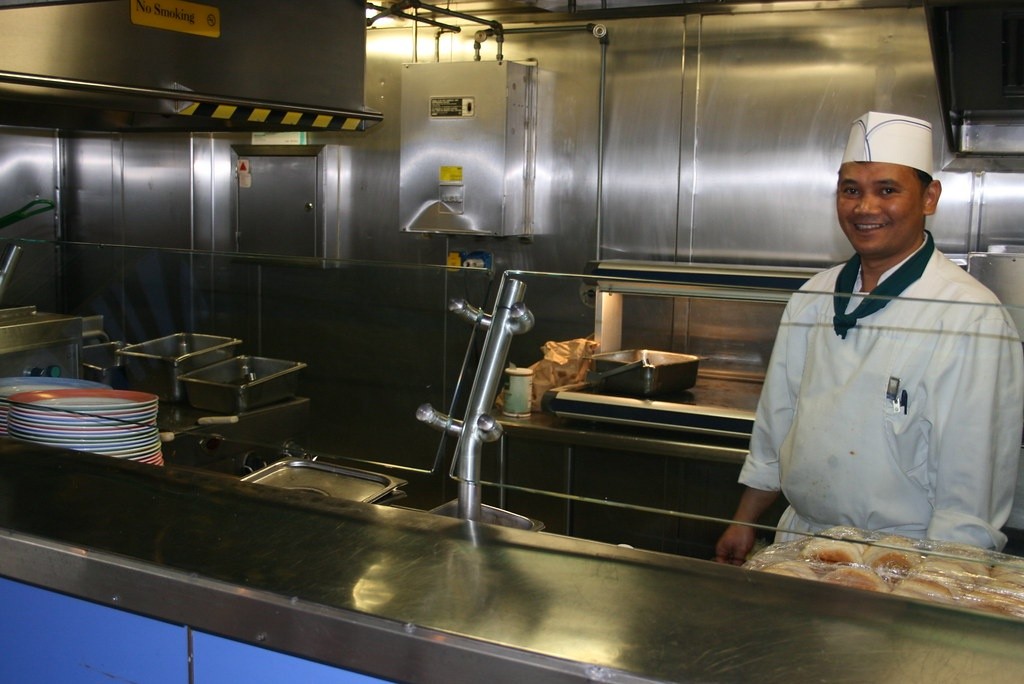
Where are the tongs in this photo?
[584,358,648,382]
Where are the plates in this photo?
[0,377,164,467]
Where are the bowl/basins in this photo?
[584,347,707,395]
[428,499,545,533]
[84,332,306,413]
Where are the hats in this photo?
[841,111,933,175]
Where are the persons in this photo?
[717,111,1024,567]
